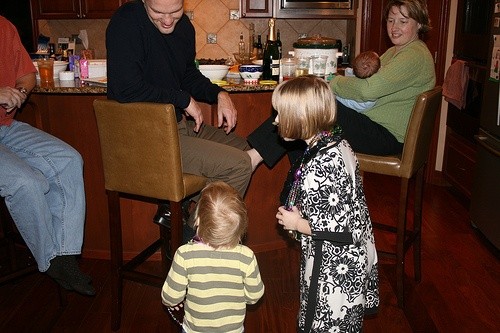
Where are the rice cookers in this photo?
[288,34,344,75]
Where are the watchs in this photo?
[16,87,28,95]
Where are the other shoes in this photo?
[45,261,89,289]
[52,255,95,296]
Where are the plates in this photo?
[253,60,263,65]
[81,77,107,87]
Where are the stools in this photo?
[92,99,209,331]
[355,85,443,310]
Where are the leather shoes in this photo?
[155,202,193,236]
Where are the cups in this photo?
[59,71,74,81]
[37,58,54,83]
[281,54,328,81]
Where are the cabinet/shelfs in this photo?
[34,0,132,20]
[241,0,357,18]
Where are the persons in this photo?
[0,15,95,295]
[106,0,252,260]
[161,181,265,333]
[335,51,381,111]
[271,74,380,333]
[244,0,436,175]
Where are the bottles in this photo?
[239,18,283,81]
[49,42,70,71]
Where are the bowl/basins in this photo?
[239,64,263,82]
[234,52,256,64]
[199,64,231,81]
[33,61,69,77]
